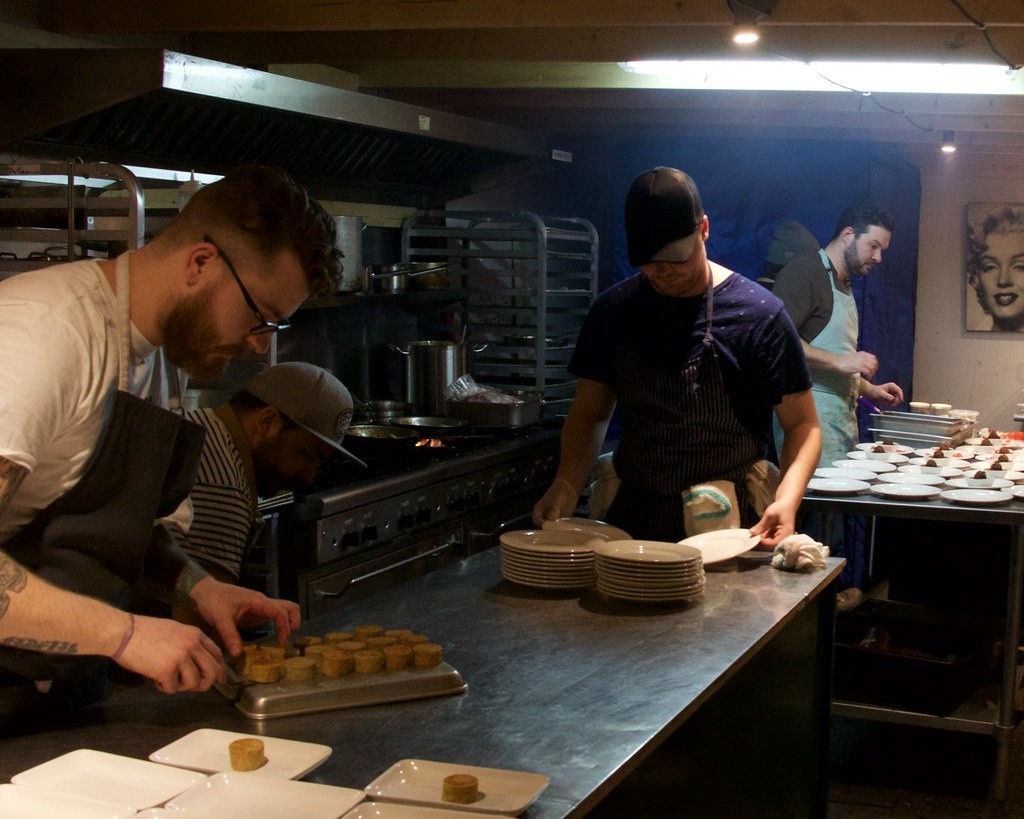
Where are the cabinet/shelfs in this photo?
[0,160,188,283]
[401,209,600,422]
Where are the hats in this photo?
[625,166,704,268]
[239,361,368,470]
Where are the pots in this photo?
[355,400,414,425]
[445,389,574,430]
[389,416,466,437]
[343,425,423,463]
[363,259,483,293]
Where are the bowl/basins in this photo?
[502,334,565,347]
[909,401,980,421]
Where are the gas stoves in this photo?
[304,395,572,516]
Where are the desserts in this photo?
[228,738,264,772]
[441,773,478,805]
[239,623,441,682]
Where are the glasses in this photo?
[204,234,292,335]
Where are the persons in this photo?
[772,199,905,617]
[168,362,368,651]
[530,165,823,818]
[0,168,345,731]
[966,202,1024,333]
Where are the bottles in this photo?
[177,168,204,213]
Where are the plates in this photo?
[807,437,1024,504]
[500,516,706,603]
[546,289,593,294]
[676,528,761,564]
[0,725,551,819]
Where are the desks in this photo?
[799,489,1023,809]
[0,556,847,819]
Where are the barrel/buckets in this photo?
[388,339,488,418]
[324,214,367,292]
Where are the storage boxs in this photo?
[446,395,540,429]
